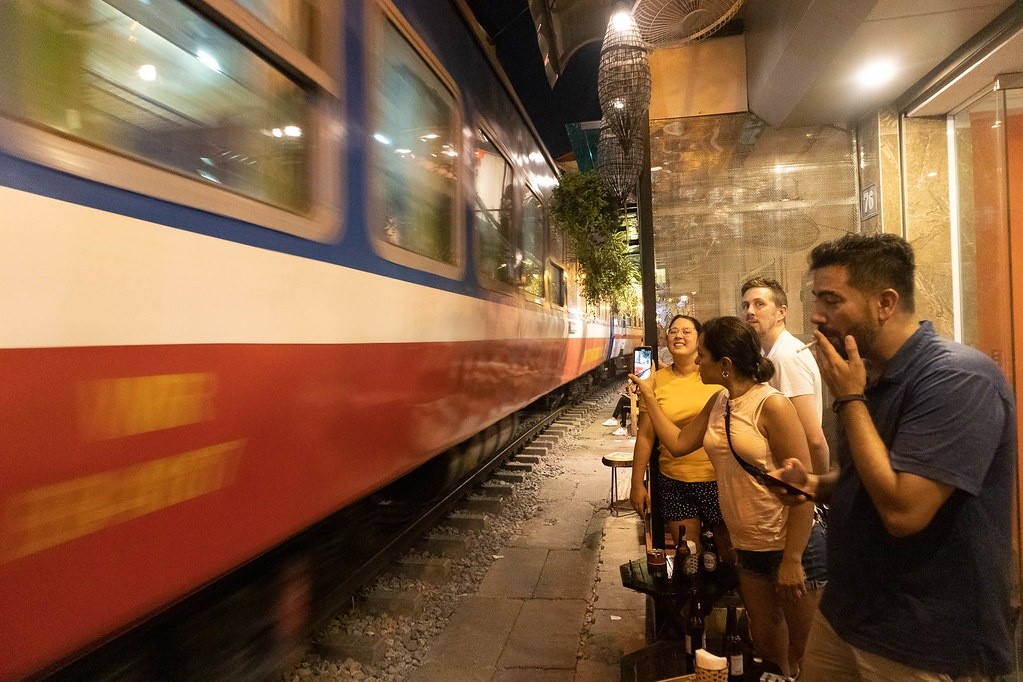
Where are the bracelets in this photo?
[833,393,870,415]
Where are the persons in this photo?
[602,378,640,436]
[768,231,1017,682]
[742,275,831,474]
[629,315,827,682]
[630,315,733,557]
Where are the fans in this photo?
[629,0,745,48]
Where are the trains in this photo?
[0,0,650,682]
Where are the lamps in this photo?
[597,7,652,209]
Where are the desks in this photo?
[602,452,650,517]
[620,556,789,682]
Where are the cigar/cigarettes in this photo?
[796,339,818,353]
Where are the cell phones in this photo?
[633,346,653,384]
[744,466,812,500]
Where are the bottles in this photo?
[672,525,691,582]
[684,587,706,674]
[721,604,743,682]
[702,526,719,583]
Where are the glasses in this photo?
[667,327,695,336]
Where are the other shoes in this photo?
[602,418,617,426]
[612,428,628,436]
